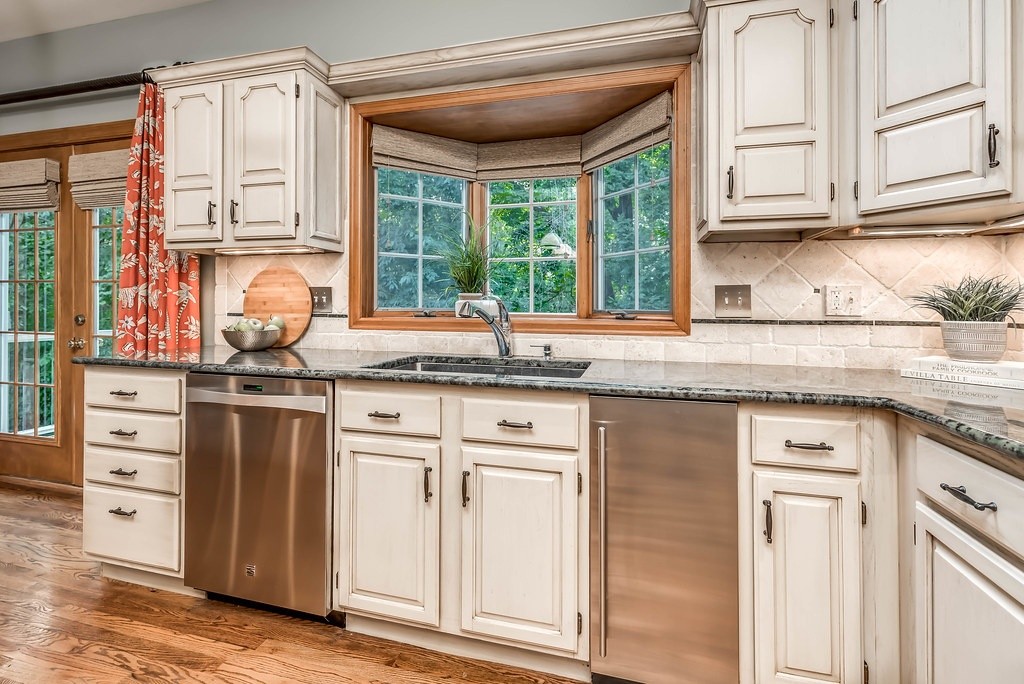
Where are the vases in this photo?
[944,402,1009,437]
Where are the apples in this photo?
[224,314,285,331]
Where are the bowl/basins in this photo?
[221,329,286,352]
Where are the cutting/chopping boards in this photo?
[243,266,313,348]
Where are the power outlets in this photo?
[308,286,332,313]
[824,284,863,317]
[715,284,751,318]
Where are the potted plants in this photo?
[906,273,1024,363]
[433,211,504,317]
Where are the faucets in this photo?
[458,302,512,356]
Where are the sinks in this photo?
[364,348,592,390]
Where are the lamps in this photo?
[540,181,562,250]
[552,205,572,255]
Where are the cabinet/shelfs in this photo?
[163,69,345,256]
[898,410,1022,683]
[739,401,899,684]
[844,0,1023,238]
[697,0,845,242]
[84,366,207,600]
[331,380,590,683]
